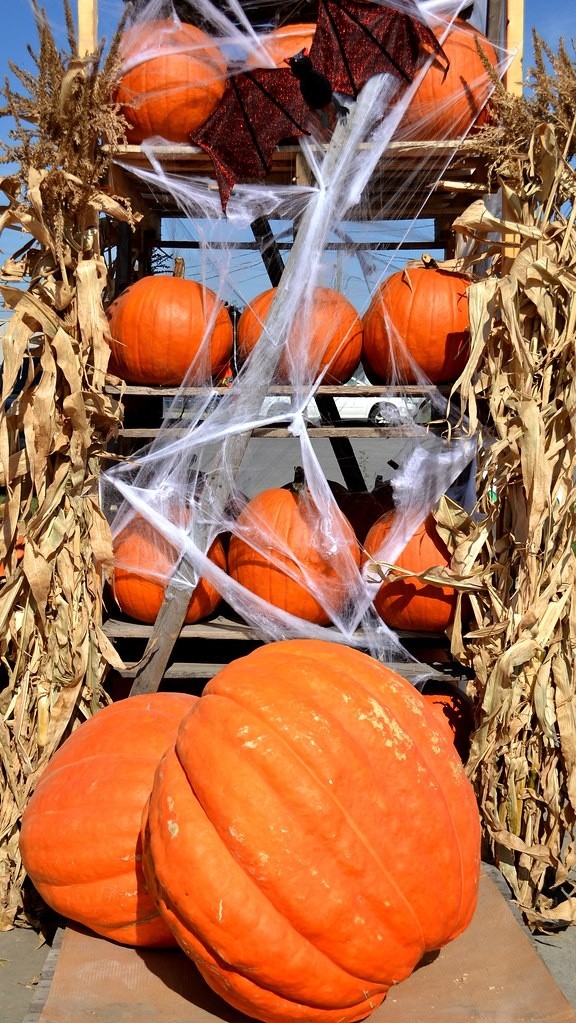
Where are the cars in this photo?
[258,376,433,428]
[163,381,223,415]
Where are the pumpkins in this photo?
[361,505,462,631]
[236,284,363,384]
[106,502,225,622]
[109,9,507,140]
[19,688,202,950]
[225,465,361,628]
[106,255,234,386]
[362,254,479,384]
[138,635,482,1023]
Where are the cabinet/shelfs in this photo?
[77,0,529,713]
